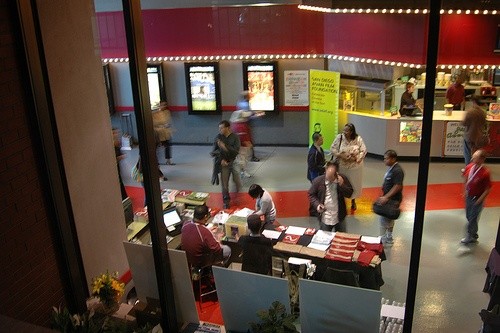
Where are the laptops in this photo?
[162,206,184,237]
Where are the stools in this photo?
[192,263,216,310]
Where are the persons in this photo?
[112,127,128,201]
[228,90,265,178]
[478,303,500,333]
[213,120,243,209]
[181,205,232,267]
[307,132,329,217]
[136,151,169,208]
[446,75,464,110]
[399,82,418,112]
[461,97,486,173]
[196,85,209,100]
[236,214,273,275]
[461,149,491,243]
[308,163,354,233]
[248,184,276,225]
[151,99,177,165]
[330,123,368,209]
[377,150,404,244]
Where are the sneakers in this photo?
[381,235,393,244]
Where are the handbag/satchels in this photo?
[323,159,339,172]
[373,199,401,219]
[480,134,490,146]
[131,159,143,183]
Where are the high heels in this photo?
[166,160,175,165]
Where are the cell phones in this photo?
[335,171,340,179]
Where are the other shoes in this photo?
[241,171,251,178]
[461,237,477,243]
[224,203,228,209]
[162,177,168,181]
[461,168,465,172]
[249,157,260,162]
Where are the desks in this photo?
[126,216,387,291]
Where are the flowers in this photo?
[90,268,125,305]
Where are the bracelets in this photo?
[355,156,356,161]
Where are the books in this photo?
[134,189,210,223]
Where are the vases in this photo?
[102,303,113,313]
